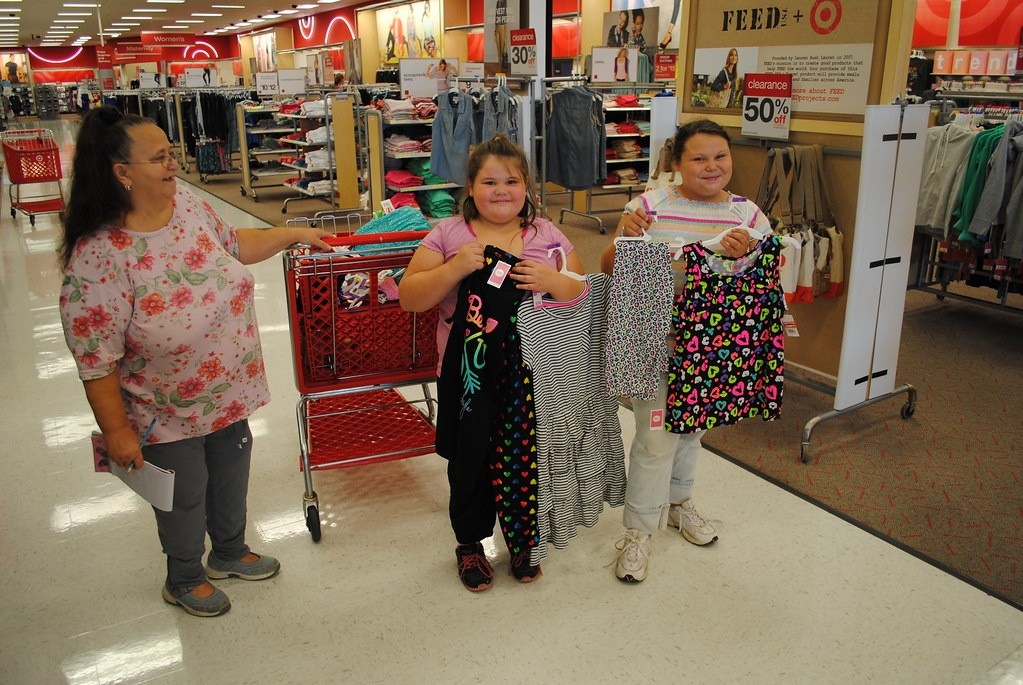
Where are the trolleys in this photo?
[287,211,462,544]
[0,128,69,226]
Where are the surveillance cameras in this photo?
[257,15,262,18]
[243,19,248,22]
[292,5,297,8]
[22,45,26,48]
[36,36,41,38]
[274,10,278,14]
[230,23,234,26]
[10,14,15,17]
[204,31,207,33]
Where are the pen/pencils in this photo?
[127,419,157,473]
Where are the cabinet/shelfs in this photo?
[601,105,652,189]
[236,102,302,177]
[367,111,468,222]
[274,113,366,195]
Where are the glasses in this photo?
[121,152,178,168]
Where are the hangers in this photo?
[565,76,603,102]
[612,212,686,246]
[350,83,398,92]
[431,74,523,106]
[674,200,800,261]
[115,89,252,100]
[949,108,1023,132]
[536,247,587,302]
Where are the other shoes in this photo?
[162,580,231,617]
[205,549,280,581]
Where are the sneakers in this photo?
[456,543,494,592]
[508,551,543,582]
[667,498,718,546]
[602,529,651,583]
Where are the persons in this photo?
[494,0,509,73]
[154,72,161,87]
[710,48,738,109]
[607,9,647,47]
[659,0,681,49]
[135,79,139,88]
[9,87,23,113]
[334,73,344,86]
[399,132,586,592]
[5,54,19,83]
[906,59,931,97]
[203,68,210,86]
[601,119,773,583]
[116,79,121,89]
[59,105,339,618]
[390,1,437,58]
[614,48,629,81]
[428,58,458,90]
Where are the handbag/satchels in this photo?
[754,143,844,305]
